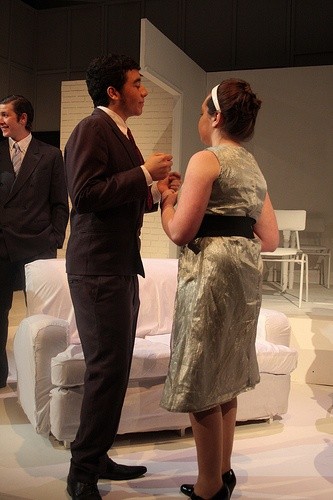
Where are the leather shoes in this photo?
[100,454,147,480]
[67,474,102,500]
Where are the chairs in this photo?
[258,209,332,309]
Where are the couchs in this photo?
[13,258,299,450]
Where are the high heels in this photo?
[181,469,236,500]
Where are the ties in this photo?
[12,143,22,180]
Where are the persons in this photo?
[64,53,184,500]
[0,95,69,390]
[159,78,280,500]
